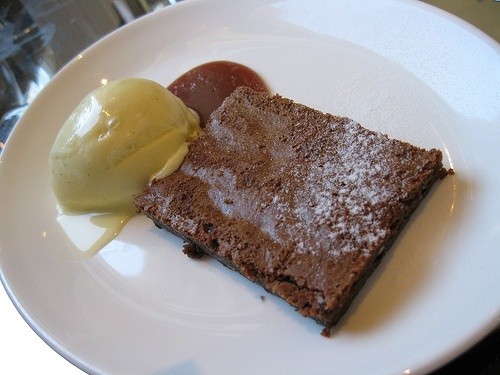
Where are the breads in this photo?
[132,86,455,339]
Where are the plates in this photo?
[0,1,500,375]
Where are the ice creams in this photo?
[48,76,201,216]
